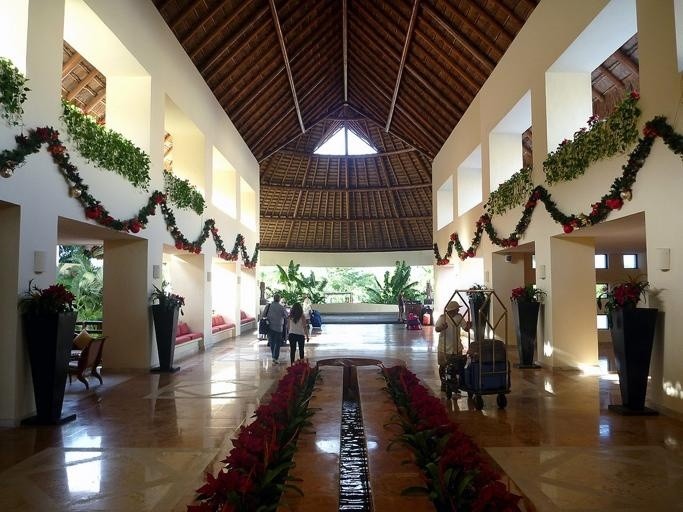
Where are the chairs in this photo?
[68,337,107,393]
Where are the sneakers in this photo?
[272,359,280,367]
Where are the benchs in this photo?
[240,309,255,334]
[210,314,233,343]
[172,322,202,360]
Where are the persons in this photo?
[398,290,406,322]
[263,294,313,366]
[435,301,472,393]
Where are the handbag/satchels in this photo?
[259,304,272,334]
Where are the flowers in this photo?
[586,276,650,308]
[378,355,530,508]
[186,350,322,511]
[430,110,681,267]
[16,279,74,312]
[0,122,261,273]
[462,282,490,300]
[149,282,185,305]
[508,280,547,304]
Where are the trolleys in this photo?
[443,287,512,412]
[265,313,288,347]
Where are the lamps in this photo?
[206,272,211,284]
[152,264,158,280]
[653,247,672,270]
[31,246,46,276]
[537,264,547,281]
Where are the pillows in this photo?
[70,328,93,349]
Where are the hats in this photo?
[447,301,463,312]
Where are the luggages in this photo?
[309,309,322,328]
[420,305,435,325]
[466,338,506,363]
[464,360,512,391]
